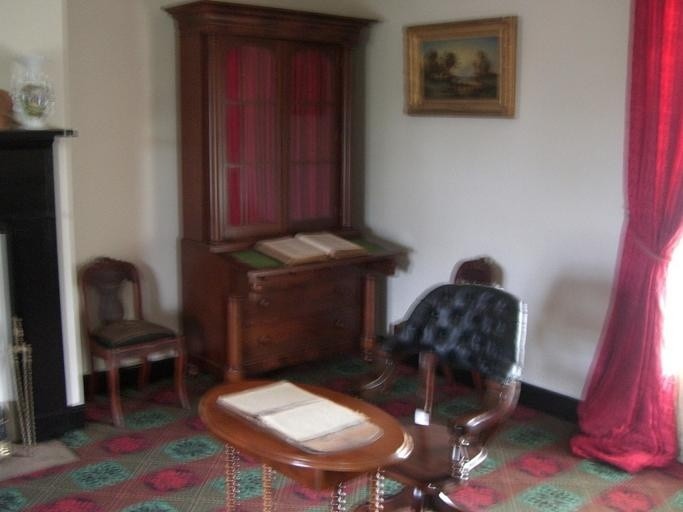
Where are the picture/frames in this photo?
[403,14,519,118]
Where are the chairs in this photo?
[77,256,191,428]
[356,283,528,512]
[438,255,506,392]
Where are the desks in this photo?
[198,379,414,512]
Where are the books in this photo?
[217,380,369,443]
[255,232,370,265]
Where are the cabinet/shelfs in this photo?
[165,0,403,388]
